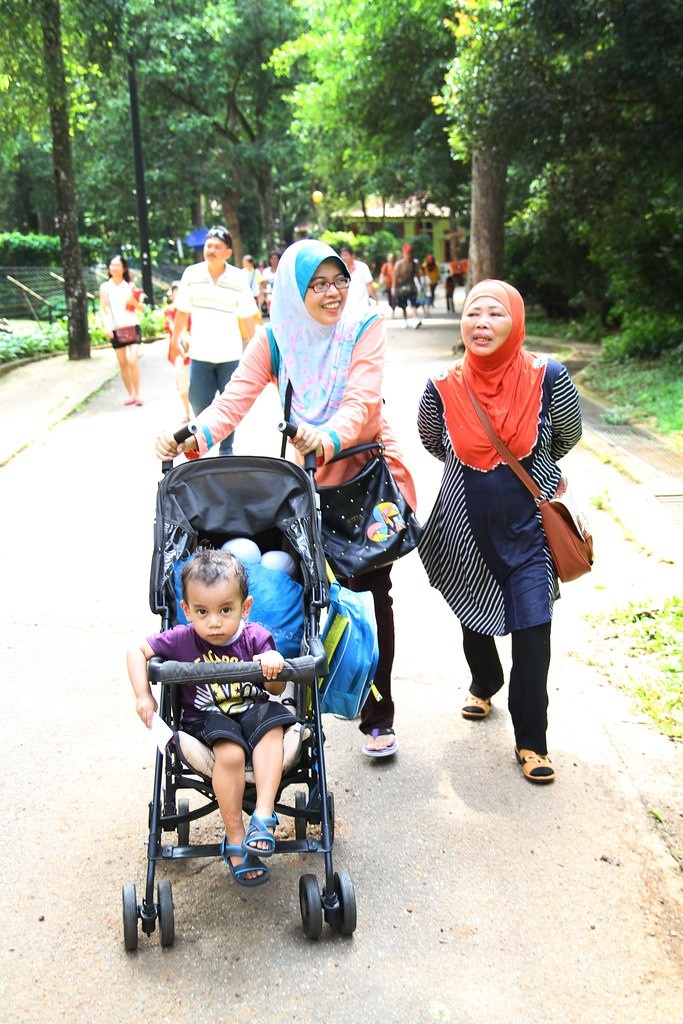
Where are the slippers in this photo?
[362,728,398,758]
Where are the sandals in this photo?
[219,834,269,887]
[462,693,491,717]
[515,746,558,783]
[241,809,279,856]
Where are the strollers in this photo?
[121,422,357,952]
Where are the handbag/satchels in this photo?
[396,279,413,296]
[316,442,423,579]
[116,326,138,343]
[539,473,593,582]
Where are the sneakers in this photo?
[413,319,422,330]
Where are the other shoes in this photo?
[124,397,134,405]
[135,397,143,406]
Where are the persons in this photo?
[416,279,582,784]
[342,245,379,306]
[157,240,416,756]
[379,245,455,328]
[127,549,303,886]
[168,227,264,455]
[164,283,191,423]
[100,256,144,407]
[243,251,280,316]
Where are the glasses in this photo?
[308,276,351,294]
